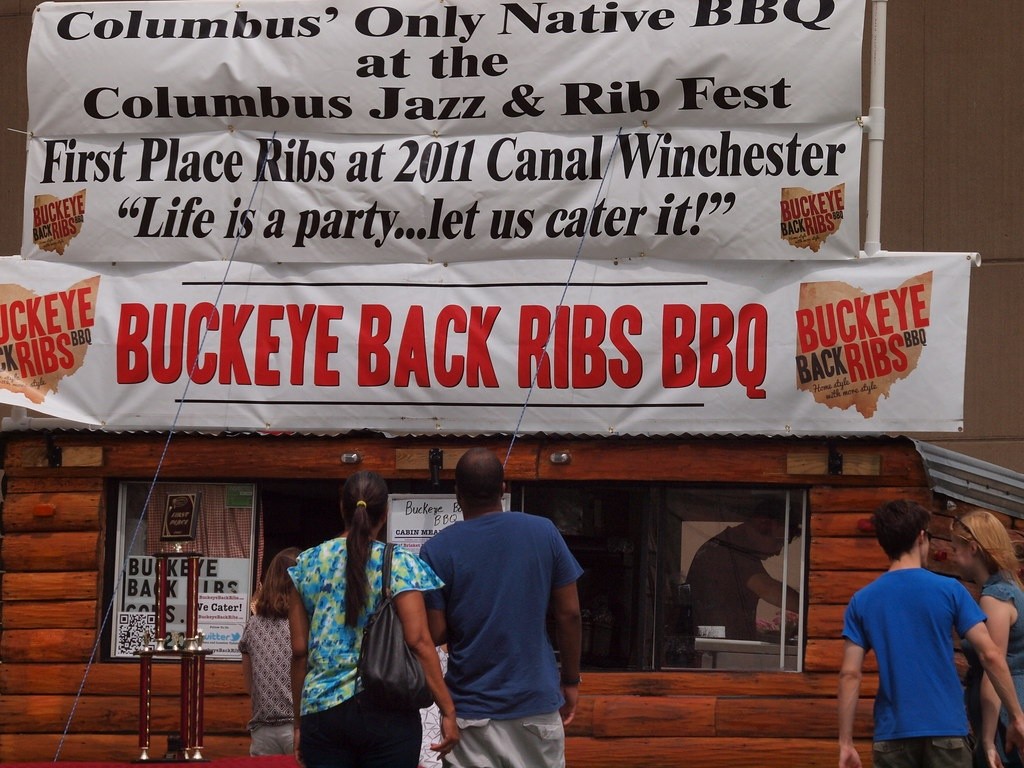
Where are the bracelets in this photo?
[561,674,582,686]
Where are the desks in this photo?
[695,637,797,671]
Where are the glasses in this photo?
[924,529,932,540]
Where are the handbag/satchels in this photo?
[356,543,434,709]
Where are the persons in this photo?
[420,448,583,768]
[289,471,460,767]
[682,515,799,666]
[237,546,301,757]
[838,501,1024,767]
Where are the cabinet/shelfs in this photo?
[545,487,648,660]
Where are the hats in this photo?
[739,493,802,522]
